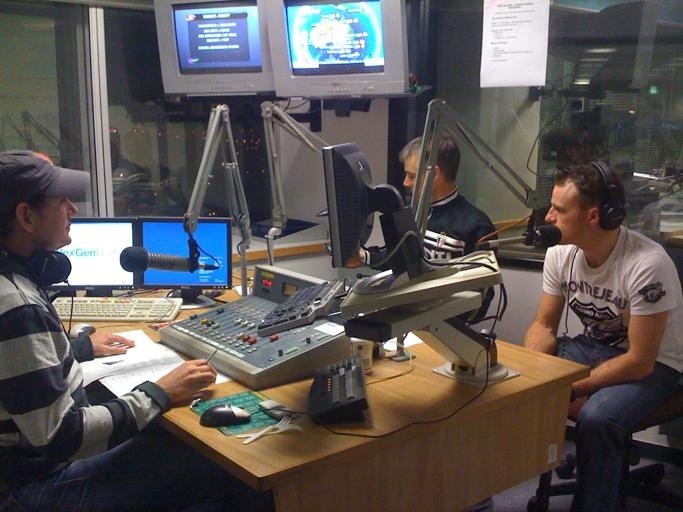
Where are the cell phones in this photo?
[260,398,292,418]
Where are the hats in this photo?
[1,149,91,202]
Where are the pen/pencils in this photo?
[205,348,219,366]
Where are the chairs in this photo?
[525,235,682,511]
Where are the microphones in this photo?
[120,245,221,273]
[477,223,562,249]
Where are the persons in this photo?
[523,160,683,512]
[343,130,498,325]
[0,146,218,512]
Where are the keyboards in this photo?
[256,277,343,338]
[51,294,183,322]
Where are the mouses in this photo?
[66,322,96,339]
[199,403,249,426]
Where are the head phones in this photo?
[1,250,71,287]
[591,159,626,232]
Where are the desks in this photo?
[44,264,592,512]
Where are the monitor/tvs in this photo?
[266,0,426,101]
[320,142,442,295]
[51,216,137,298]
[137,217,233,308]
[155,0,276,102]
